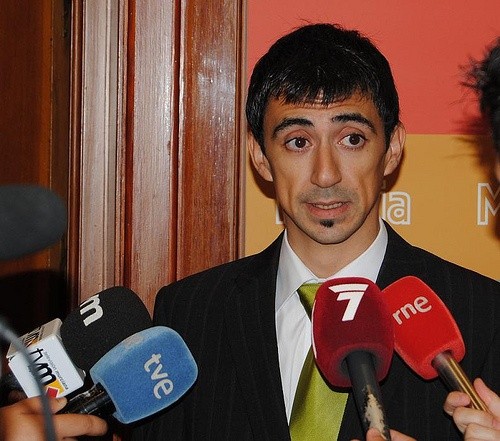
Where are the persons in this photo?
[443,36,500,441]
[122,19,500,441]
[351,427,420,441]
[0,389,120,441]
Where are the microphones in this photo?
[312,275,393,441]
[53,326,200,423]
[2,287,153,404]
[379,274,493,425]
